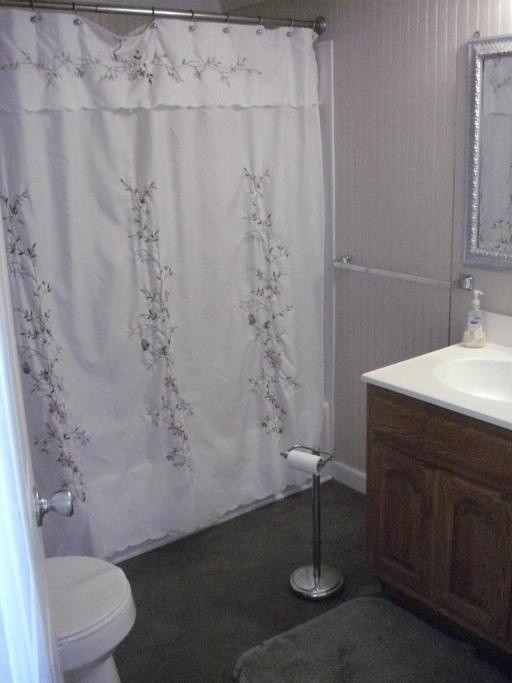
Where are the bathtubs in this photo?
[41,402,331,565]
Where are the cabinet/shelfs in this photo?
[364,380,512,662]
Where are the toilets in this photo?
[46,556,137,683]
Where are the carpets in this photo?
[232,595,499,683]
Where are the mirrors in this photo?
[463,33,512,270]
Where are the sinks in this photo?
[433,357,512,401]
